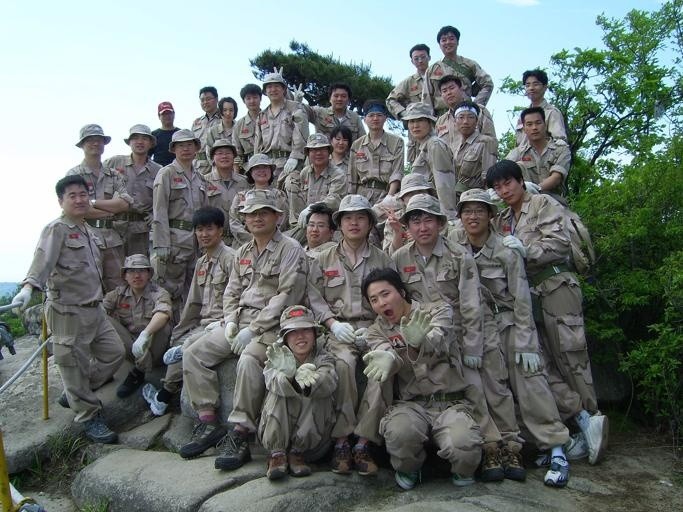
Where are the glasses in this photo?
[460,208,488,215]
[306,147,329,153]
[455,114,476,122]
[213,152,235,156]
[173,142,197,149]
[200,97,215,102]
[306,223,329,228]
[247,210,270,217]
[125,268,144,273]
[524,83,542,87]
[367,112,384,117]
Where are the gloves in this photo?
[330,320,356,344]
[225,322,238,346]
[295,363,319,390]
[400,308,434,348]
[485,188,504,203]
[284,158,298,176]
[205,321,222,334]
[298,204,315,229]
[112,191,121,200]
[524,181,541,194]
[273,67,284,78]
[463,354,482,370]
[502,235,527,258]
[516,353,540,373]
[293,83,305,102]
[144,211,153,227]
[156,248,170,261]
[399,110,407,117]
[354,328,369,351]
[231,326,256,356]
[264,342,296,381]
[448,218,464,229]
[132,330,149,359]
[362,349,397,383]
[12,288,32,315]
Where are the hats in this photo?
[398,173,437,199]
[277,304,323,344]
[169,129,201,153]
[399,192,447,226]
[75,124,111,147]
[455,188,498,218]
[243,154,276,175]
[262,73,287,96]
[332,194,377,226]
[401,102,437,122]
[124,124,157,147]
[304,133,333,155]
[120,254,154,281]
[238,189,284,213]
[158,102,174,115]
[210,139,237,160]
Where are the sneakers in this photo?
[117,367,144,398]
[328,440,354,474]
[352,447,378,476]
[58,390,70,408]
[582,415,608,466]
[452,473,475,486]
[475,442,505,482]
[163,345,183,365]
[84,410,118,444]
[534,454,551,467]
[566,409,602,461]
[544,452,569,488]
[287,451,311,477]
[180,417,227,460]
[501,441,527,481]
[266,454,288,479]
[395,470,422,490]
[92,376,113,392]
[142,383,168,417]
[215,430,250,470]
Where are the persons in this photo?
[13,174,125,444]
[385,44,432,121]
[66,65,609,492]
[421,26,494,116]
